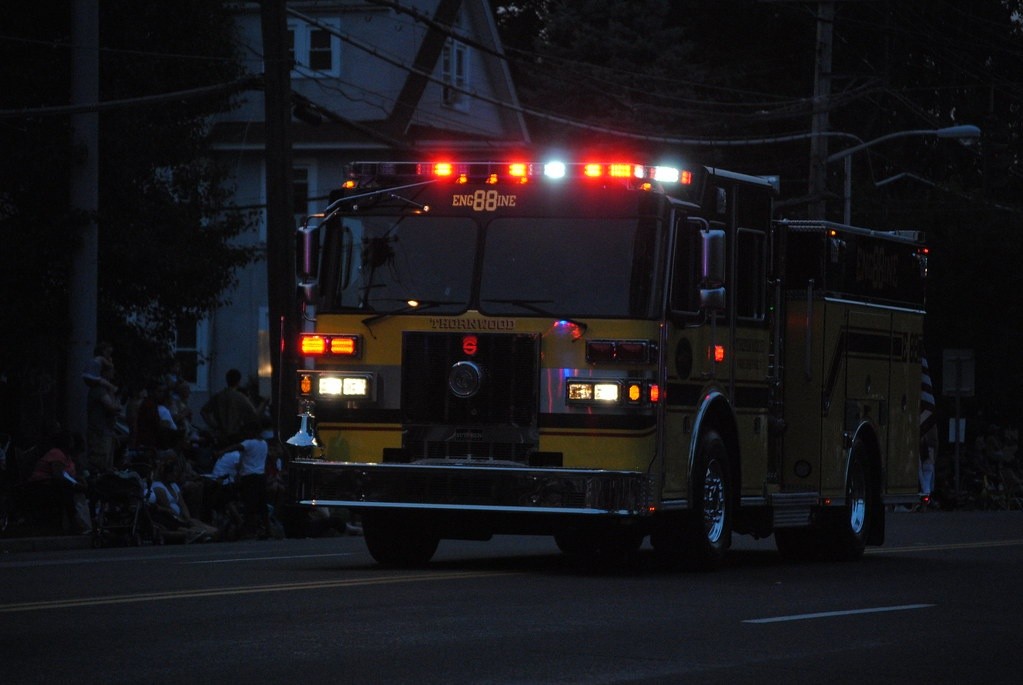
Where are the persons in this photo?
[894,441,1023,511]
[0,335,364,546]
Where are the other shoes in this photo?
[184,527,207,544]
[76,527,93,535]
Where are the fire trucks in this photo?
[284,141,927,579]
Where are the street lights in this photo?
[804,125,982,221]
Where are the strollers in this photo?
[92,461,164,548]
